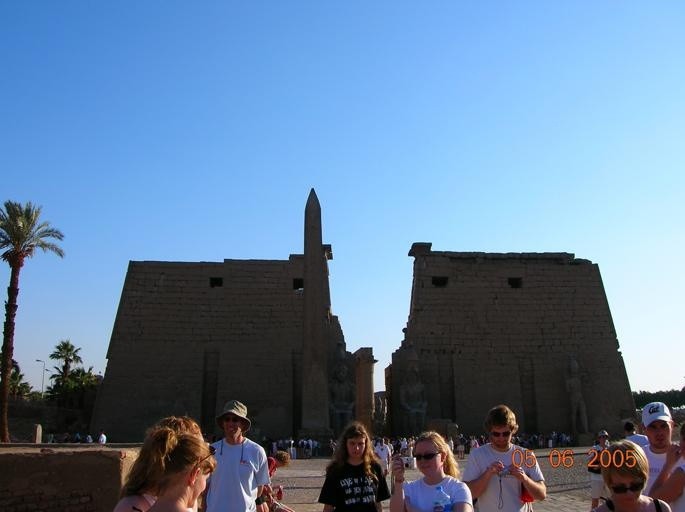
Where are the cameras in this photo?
[499,464,517,476]
[399,457,416,469]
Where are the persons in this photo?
[48,428,107,444]
[511,431,572,449]
[392,436,419,457]
[318,425,391,512]
[462,405,546,512]
[585,430,612,509]
[390,432,474,512]
[444,432,489,459]
[648,423,685,512]
[121,428,216,512]
[200,400,271,512]
[624,422,649,447]
[589,439,672,512]
[257,451,290,512]
[370,434,394,477]
[114,416,204,512]
[641,402,674,496]
[261,434,338,459]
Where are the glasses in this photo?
[414,452,442,461]
[196,445,217,467]
[608,480,644,494]
[491,430,512,437]
[223,415,241,422]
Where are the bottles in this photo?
[432,487,450,512]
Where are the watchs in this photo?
[395,477,404,482]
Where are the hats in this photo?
[642,402,672,428]
[216,399,251,433]
[598,430,608,436]
